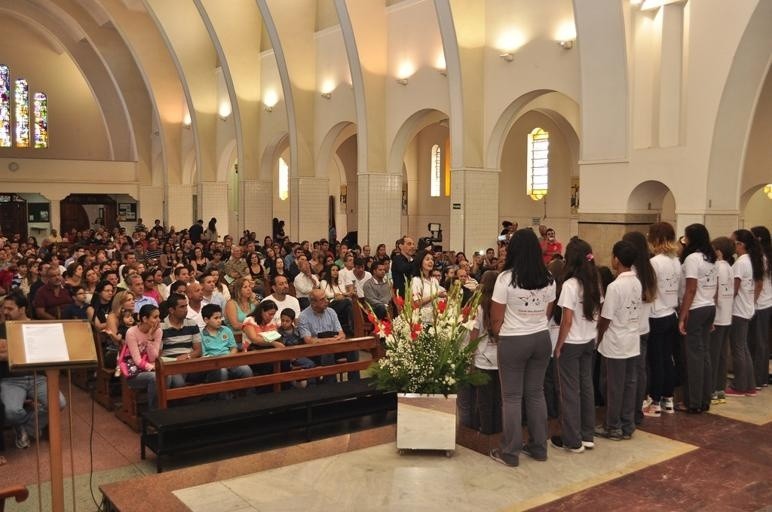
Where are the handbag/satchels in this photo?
[114,344,152,378]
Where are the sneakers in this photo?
[592,428,620,440]
[710,394,719,404]
[723,387,747,398]
[641,401,663,418]
[582,440,595,450]
[520,442,544,461]
[550,434,584,454]
[12,424,32,449]
[745,387,758,396]
[763,383,769,387]
[660,397,675,414]
[686,407,701,414]
[716,390,726,404]
[755,386,761,390]
[701,404,710,410]
[622,430,632,441]
[490,447,517,468]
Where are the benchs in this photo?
[1,227,396,511]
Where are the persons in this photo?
[294,234,505,332]
[0,216,361,465]
[485,217,771,465]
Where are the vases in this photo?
[395,392,458,459]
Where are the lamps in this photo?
[263,107,273,113]
[498,53,513,62]
[394,79,408,85]
[560,40,573,49]
[321,90,331,99]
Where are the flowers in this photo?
[344,268,493,401]
